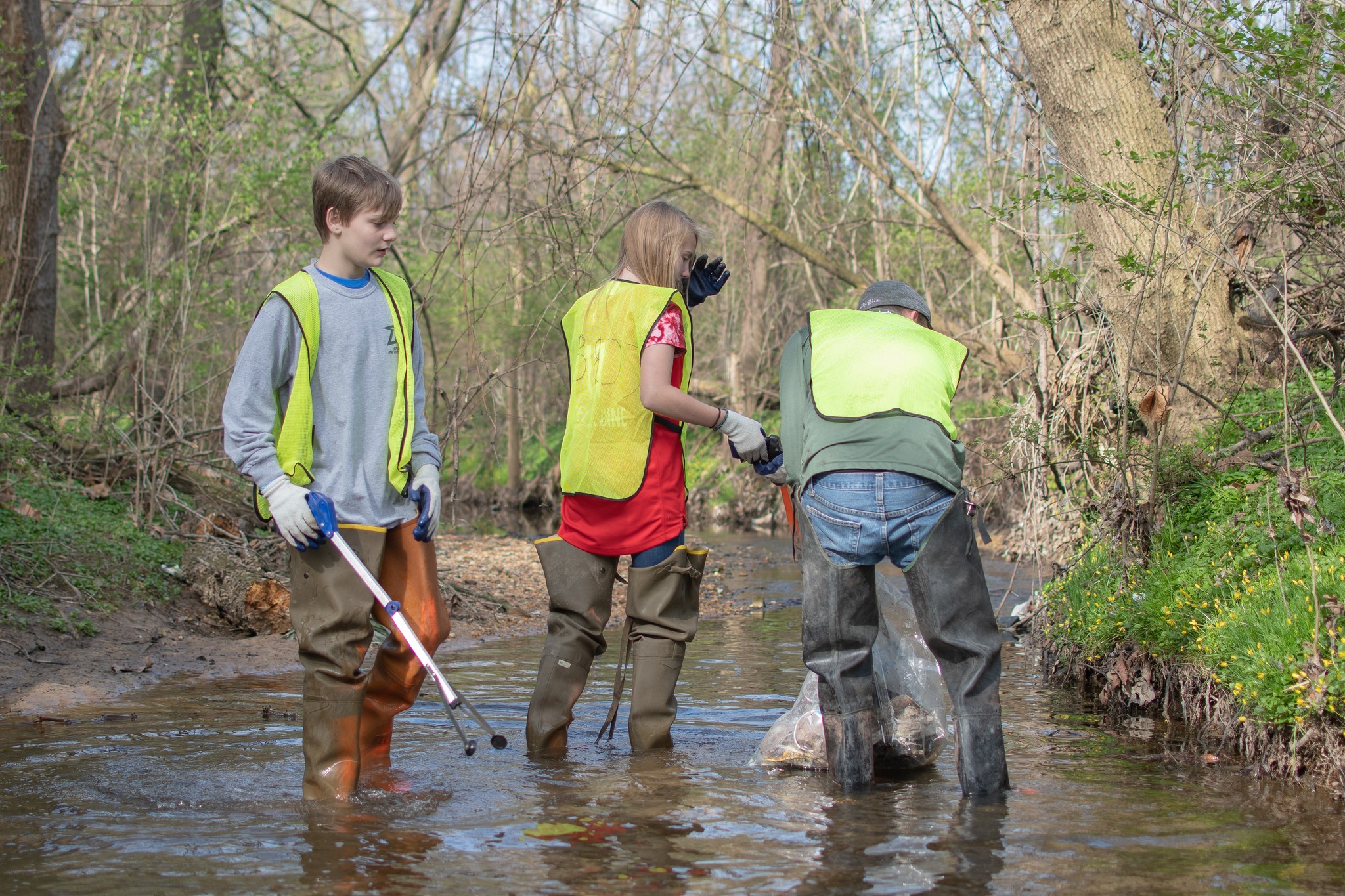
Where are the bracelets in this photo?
[709,407,721,429]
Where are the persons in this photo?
[220,155,446,799]
[775,280,1013,799]
[527,201,772,758]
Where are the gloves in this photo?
[408,462,441,542]
[261,474,326,551]
[713,408,768,464]
[682,254,730,307]
[753,434,788,487]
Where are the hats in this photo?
[857,281,933,330]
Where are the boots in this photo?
[902,490,1010,800]
[793,487,879,794]
[285,523,386,799]
[525,534,619,763]
[365,518,451,758]
[596,545,708,751]
